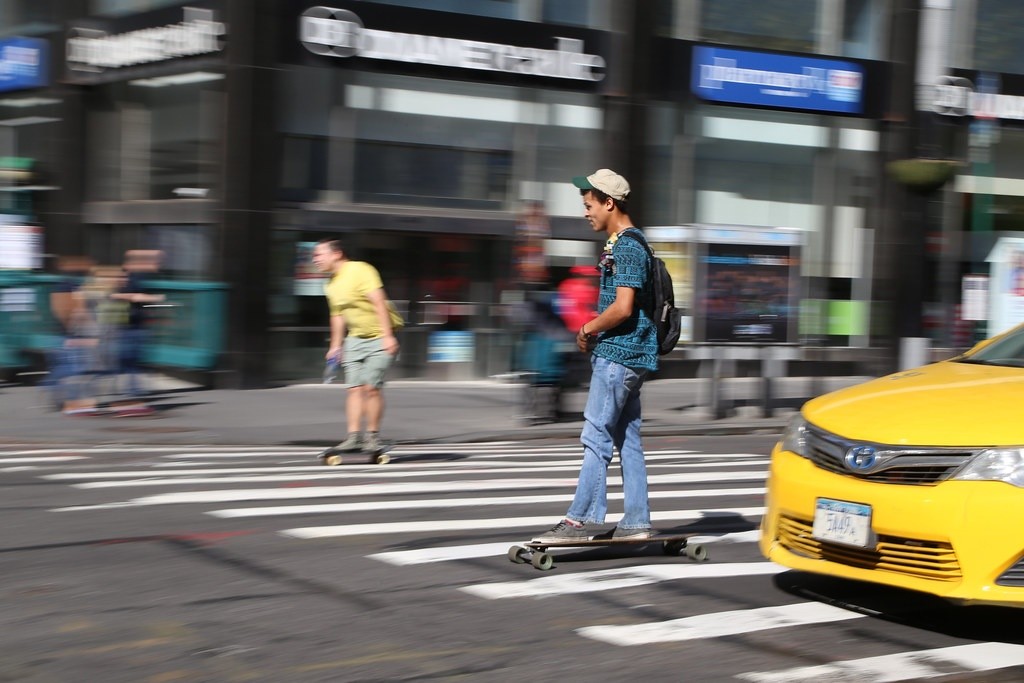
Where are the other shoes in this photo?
[362,432,388,452]
[333,434,363,450]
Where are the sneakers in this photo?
[531,520,595,543]
[592,526,653,541]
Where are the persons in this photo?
[532,169,659,543]
[46,249,166,419]
[312,238,404,451]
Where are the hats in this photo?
[572,168,633,202]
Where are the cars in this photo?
[760,322,1023,610]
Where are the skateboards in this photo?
[507,533,708,571]
[315,446,397,466]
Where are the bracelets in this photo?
[582,324,586,336]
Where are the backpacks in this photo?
[600,230,681,356]
